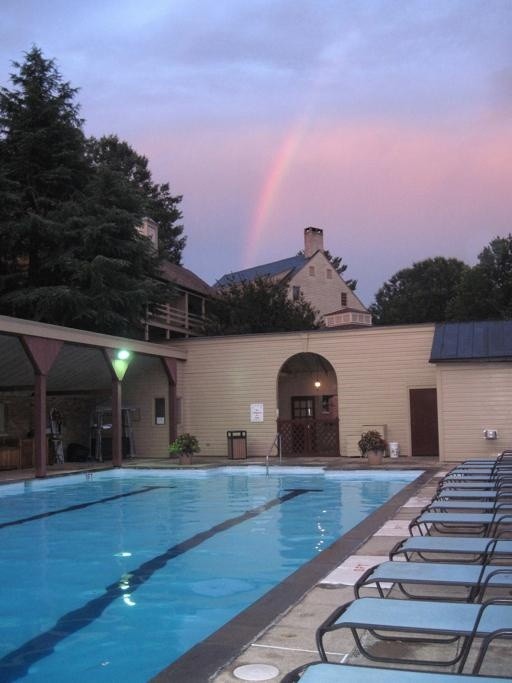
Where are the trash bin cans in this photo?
[227,431,248,459]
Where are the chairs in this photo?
[431,449,511,529]
[314,569,511,674]
[420,480,512,535]
[279,628,511,683]
[407,492,511,563]
[354,536,512,644]
[387,515,511,602]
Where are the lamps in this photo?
[313,357,321,391]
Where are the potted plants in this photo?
[165,432,201,466]
[357,430,390,465]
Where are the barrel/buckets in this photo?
[388,442,399,458]
